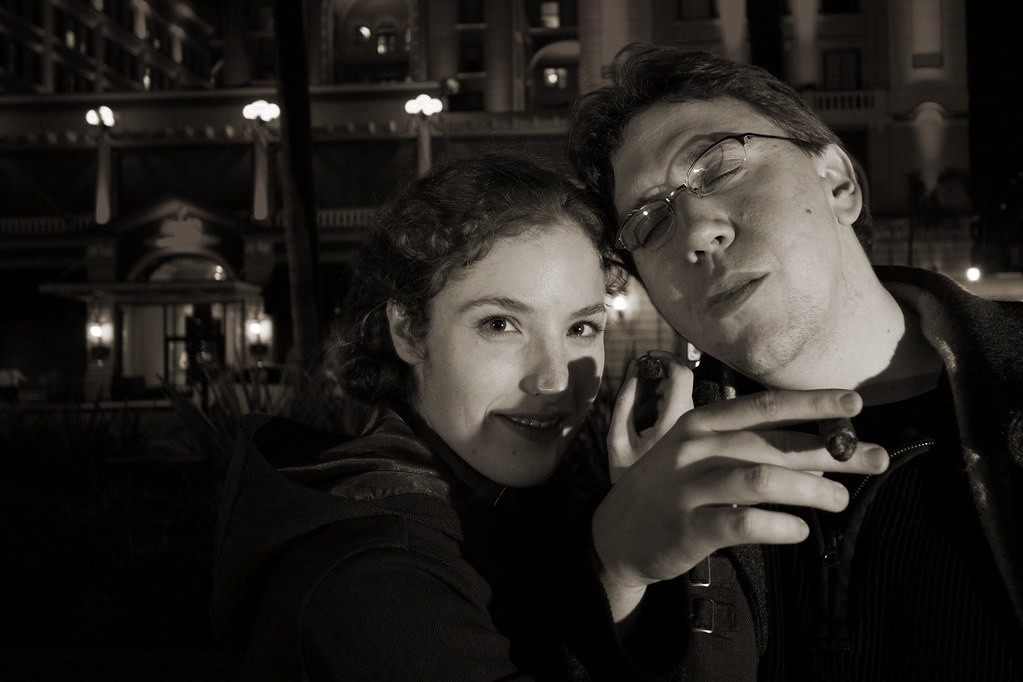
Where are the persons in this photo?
[212,43,1023,682]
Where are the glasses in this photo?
[618,131,822,254]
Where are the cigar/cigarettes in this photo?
[638,358,661,380]
[818,418,858,462]
